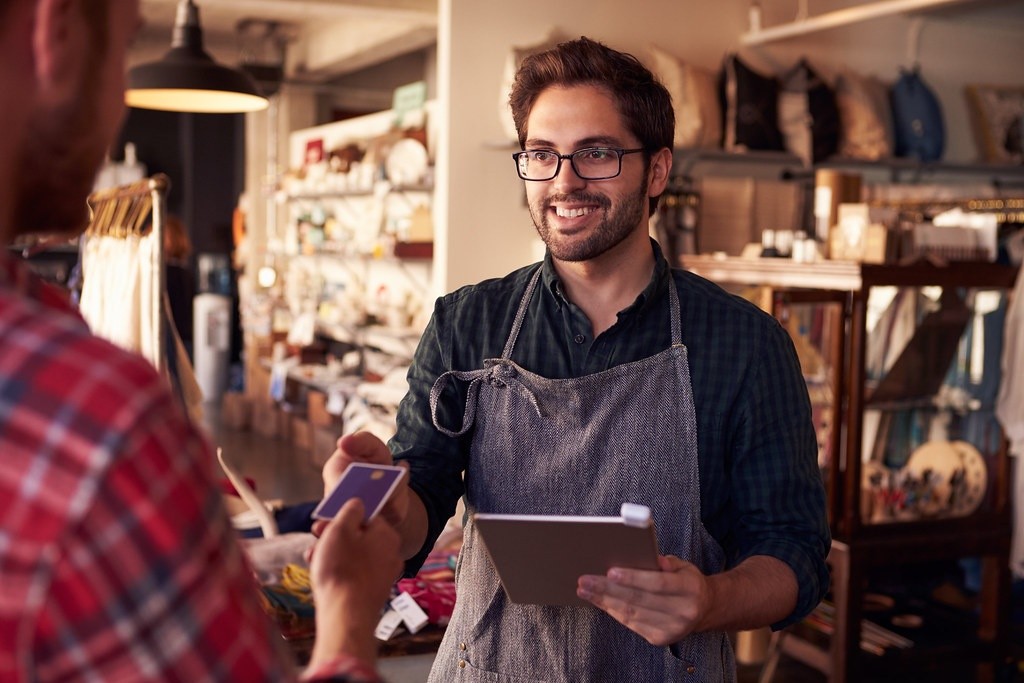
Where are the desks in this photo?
[275,617,450,683]
[181,395,339,522]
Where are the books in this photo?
[472,512,664,604]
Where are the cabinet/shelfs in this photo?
[647,149,1024,268]
[258,97,438,446]
[678,253,1023,683]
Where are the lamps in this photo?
[119,1,271,113]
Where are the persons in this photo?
[0,0,401,683]
[323,36,830,682]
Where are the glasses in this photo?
[513,147,646,181]
